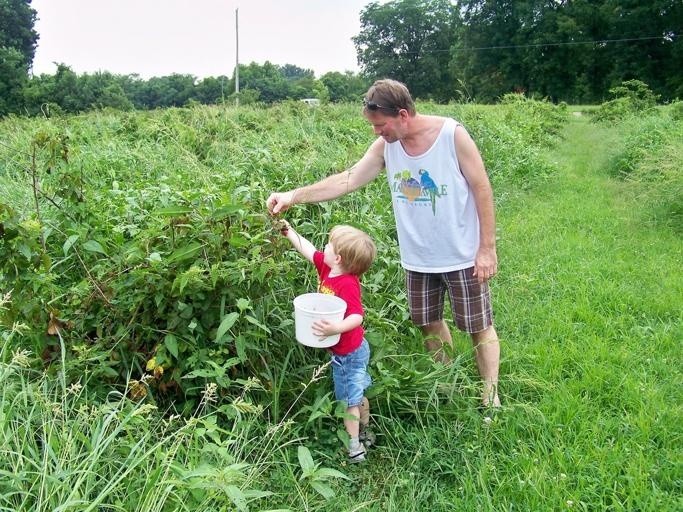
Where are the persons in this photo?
[277,215,378,464]
[265,76,502,426]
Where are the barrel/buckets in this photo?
[293,292,348,348]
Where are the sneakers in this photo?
[333,427,377,465]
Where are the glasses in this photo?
[363,97,399,113]
